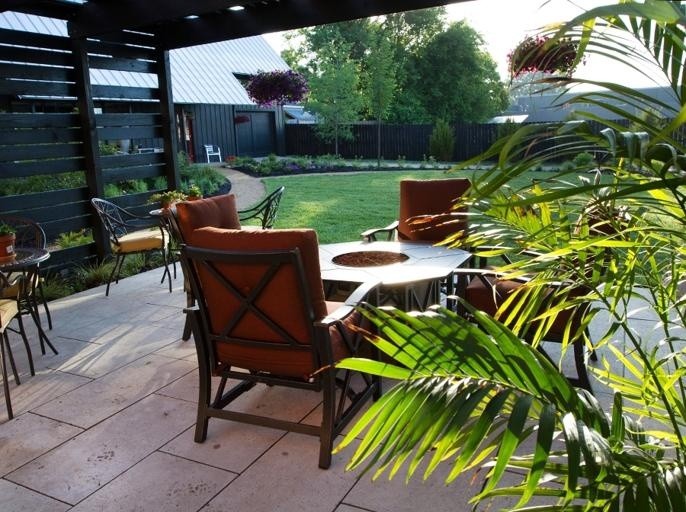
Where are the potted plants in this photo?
[145,189,188,211]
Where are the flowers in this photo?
[245,68,312,109]
[505,35,587,80]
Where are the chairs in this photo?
[0,214,58,420]
[91,197,177,297]
[202,144,222,163]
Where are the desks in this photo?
[148,208,186,293]
